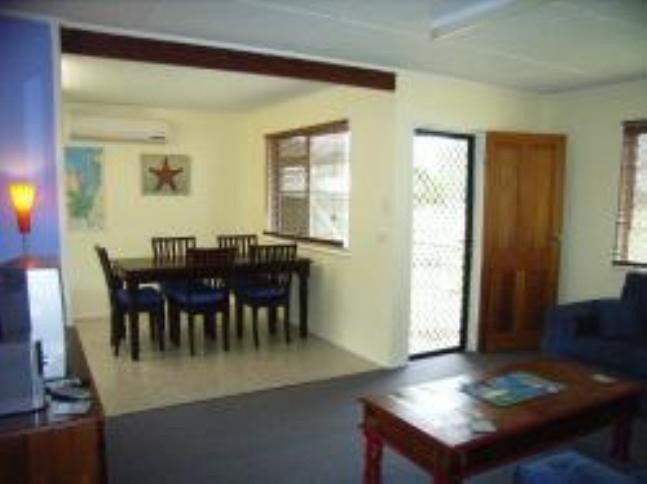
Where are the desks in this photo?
[355,358,647,484]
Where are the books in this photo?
[460,368,566,408]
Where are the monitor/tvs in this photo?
[0,254,68,417]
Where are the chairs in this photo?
[92,233,313,362]
[542,268,647,373]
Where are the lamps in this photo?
[8,179,38,237]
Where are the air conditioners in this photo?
[63,113,169,143]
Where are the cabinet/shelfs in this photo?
[1,420,110,484]
